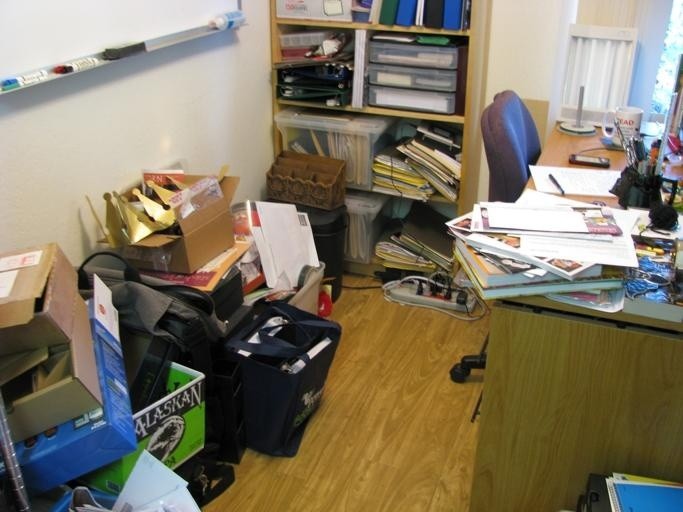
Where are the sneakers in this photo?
[187,464,235,508]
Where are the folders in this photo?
[277,69,353,101]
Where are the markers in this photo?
[0,0,242,96]
[3,71,46,86]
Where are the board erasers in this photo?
[102,42,145,59]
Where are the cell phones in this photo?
[569,155,610,167]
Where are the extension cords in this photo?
[384,281,477,312]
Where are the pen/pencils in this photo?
[2,76,44,93]
[613,118,661,177]
[548,174,565,194]
[66,61,99,72]
[54,57,95,73]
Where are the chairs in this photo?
[448,89,543,381]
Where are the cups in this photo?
[601,107,643,146]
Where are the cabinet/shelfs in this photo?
[269,0,492,279]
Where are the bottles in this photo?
[208,11,247,30]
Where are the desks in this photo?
[467,119,683,512]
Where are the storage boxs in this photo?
[273,108,398,263]
[103,173,245,321]
[0,243,204,498]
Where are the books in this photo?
[372,121,462,202]
[445,196,641,300]
[374,200,454,274]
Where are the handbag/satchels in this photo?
[76,258,225,362]
[226,299,344,456]
